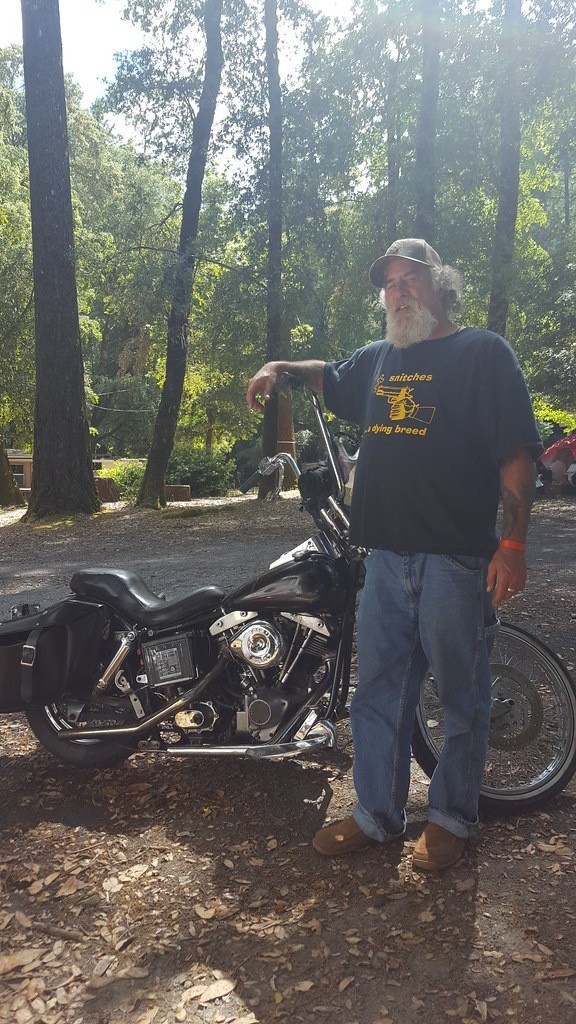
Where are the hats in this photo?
[369,238,442,288]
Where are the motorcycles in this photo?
[537,418,568,451]
[0,372,576,813]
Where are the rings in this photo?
[506,588,515,592]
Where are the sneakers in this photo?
[412,821,463,870]
[312,814,378,856]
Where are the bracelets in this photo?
[499,540,526,551]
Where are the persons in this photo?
[247,239,544,872]
